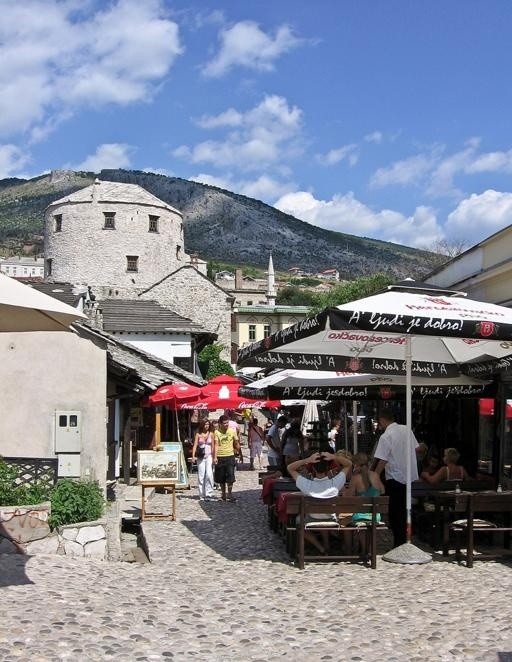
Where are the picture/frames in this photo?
[159,440,190,489]
[136,449,181,483]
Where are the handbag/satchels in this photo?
[195,445,205,459]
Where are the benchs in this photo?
[258,472,512,571]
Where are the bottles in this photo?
[455,484,460,493]
[496,484,502,494]
